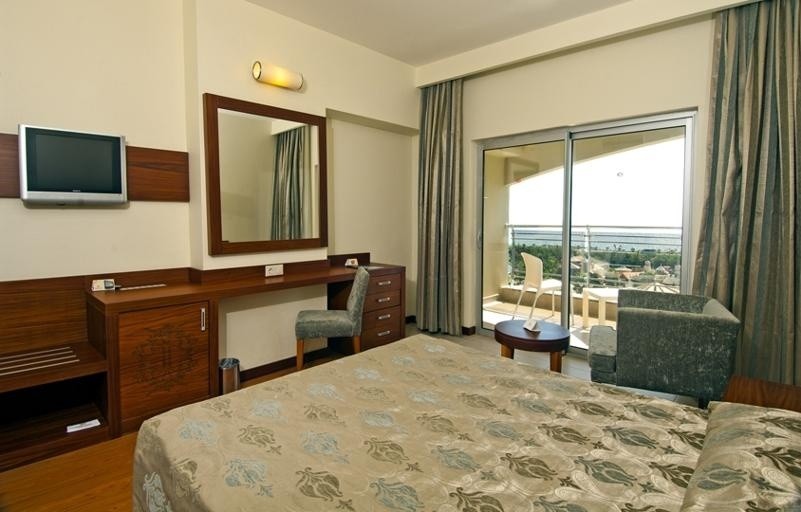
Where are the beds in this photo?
[137,333,801,512]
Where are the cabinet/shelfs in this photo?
[1,342,111,473]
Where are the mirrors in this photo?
[202,93,329,256]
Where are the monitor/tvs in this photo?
[17,122,129,207]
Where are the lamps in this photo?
[252,60,304,90]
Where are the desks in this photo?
[87,261,406,441]
[581,287,619,328]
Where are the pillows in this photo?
[678,401,801,512]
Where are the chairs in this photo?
[295,266,370,371]
[512,251,576,327]
[586,288,741,409]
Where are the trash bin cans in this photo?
[218,358,240,395]
